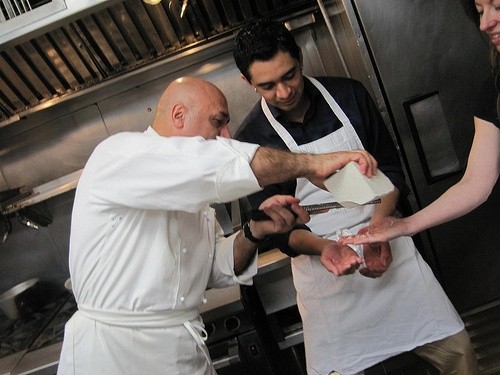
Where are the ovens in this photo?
[197,301,276,373]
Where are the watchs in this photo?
[242,222,266,243]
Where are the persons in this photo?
[339,0,500,244]
[232,19,477,375]
[53,77,379,375]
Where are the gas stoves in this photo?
[0,299,80,359]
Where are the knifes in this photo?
[250,198,381,221]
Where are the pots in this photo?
[0,277,42,318]
[64,277,72,293]
